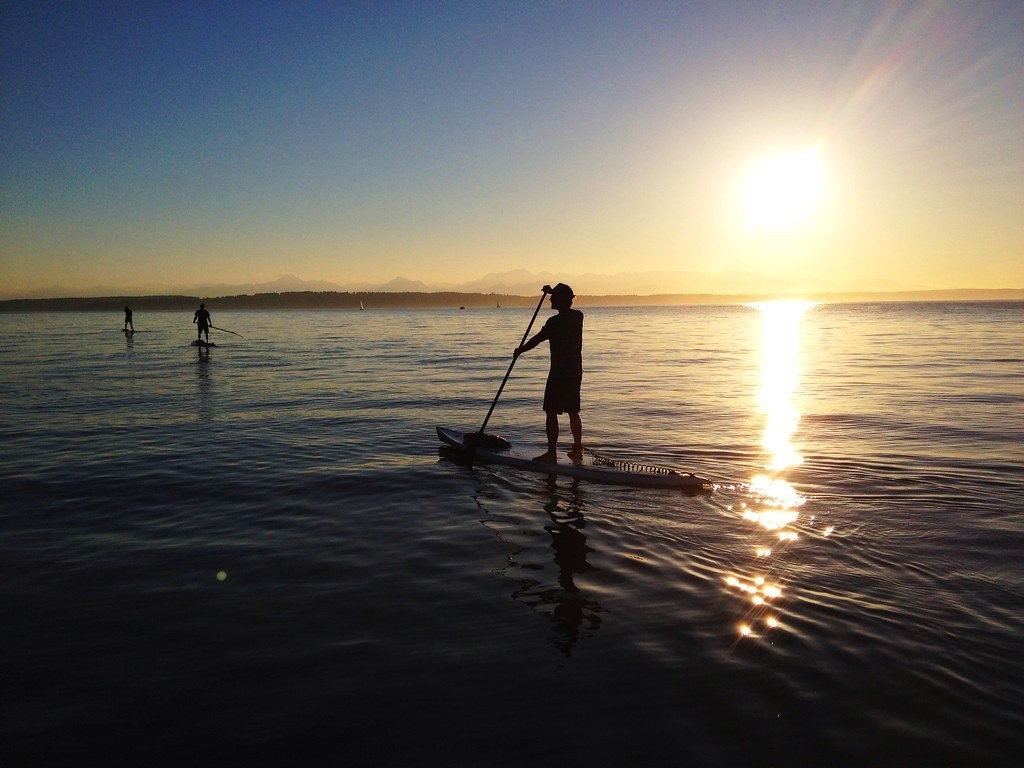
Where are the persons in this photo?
[124,306,134,331]
[512,283,584,462]
[194,304,212,343]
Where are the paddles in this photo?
[479,290,549,434]
[193,320,243,338]
